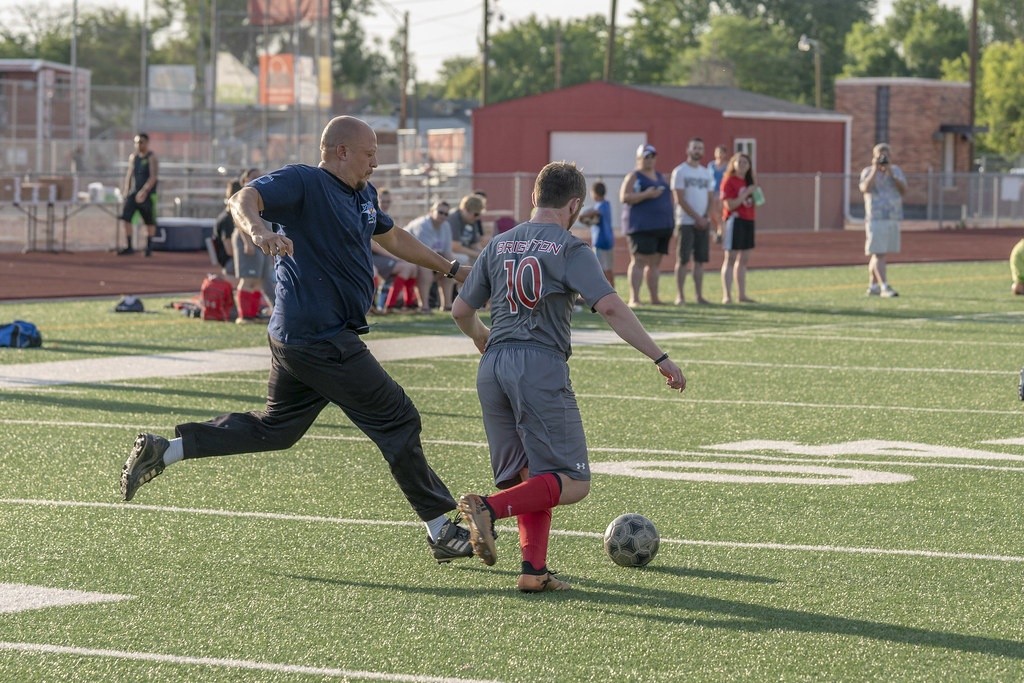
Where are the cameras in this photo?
[877,157,889,164]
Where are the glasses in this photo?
[435,210,449,217]
[468,210,481,217]
[643,153,656,159]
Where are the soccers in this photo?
[602,512,661,569]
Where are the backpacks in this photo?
[200,275,233,319]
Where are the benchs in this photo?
[145,216,217,252]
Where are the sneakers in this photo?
[120,433,170,500]
[459,494,497,566]
[426,511,475,565]
[519,560,570,592]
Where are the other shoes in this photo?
[867,285,881,294]
[880,285,899,297]
[235,316,270,325]
[144,248,152,257]
[119,247,134,255]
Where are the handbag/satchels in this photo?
[0,320,43,348]
[115,297,143,312]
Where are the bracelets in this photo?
[654,353,669,365]
[444,260,460,279]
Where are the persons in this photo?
[706,146,727,244]
[859,142,908,298]
[118,133,158,257]
[578,182,614,290]
[452,161,686,593]
[721,151,757,304]
[1010,237,1024,401]
[620,144,675,307]
[670,137,712,306]
[365,189,490,316]
[119,115,489,564]
[214,168,277,323]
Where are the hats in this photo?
[637,143,656,159]
